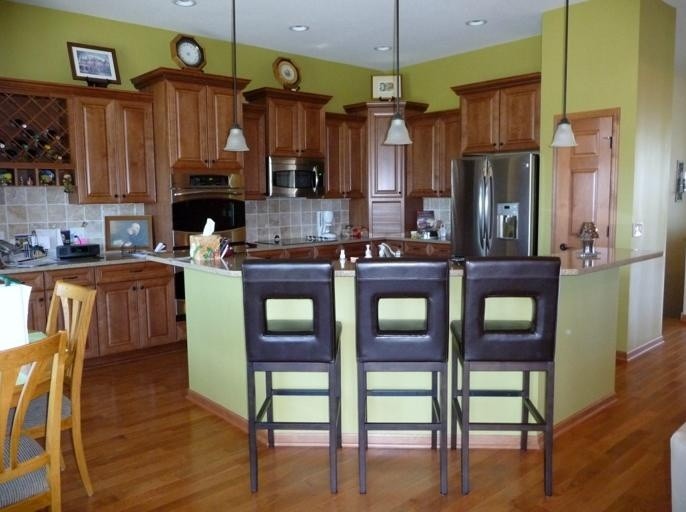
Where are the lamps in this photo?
[546,1,578,150]
[382,0,413,146]
[223,1,250,152]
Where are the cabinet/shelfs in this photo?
[68,95,156,207]
[324,117,364,199]
[3,267,99,362]
[402,110,462,198]
[250,95,326,160]
[240,106,268,196]
[457,80,539,153]
[139,79,244,172]
[251,240,450,263]
[349,107,422,199]
[96,261,177,359]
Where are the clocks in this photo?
[170,33,207,71]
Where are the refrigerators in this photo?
[451,150,538,262]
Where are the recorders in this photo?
[54,243,101,260]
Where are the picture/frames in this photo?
[66,40,123,87]
[370,72,402,101]
[103,214,154,252]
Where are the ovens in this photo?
[172,168,245,322]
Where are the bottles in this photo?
[0,117,67,164]
[341,224,368,242]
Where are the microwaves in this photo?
[266,156,325,197]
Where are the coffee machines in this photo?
[316,211,337,242]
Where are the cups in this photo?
[412,223,447,243]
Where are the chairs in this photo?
[1,278,99,512]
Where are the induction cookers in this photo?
[255,235,327,245]
